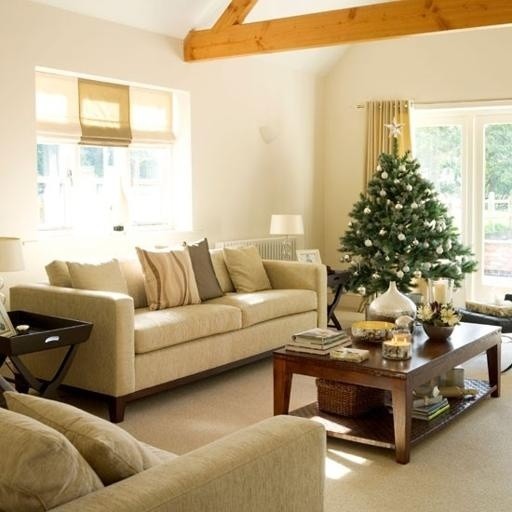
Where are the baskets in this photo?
[316,378,385,419]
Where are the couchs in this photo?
[9,239,327,426]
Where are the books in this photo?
[388,383,450,421]
[285,327,353,355]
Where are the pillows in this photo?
[1,407,104,512]
[5,389,165,480]
[45,240,272,311]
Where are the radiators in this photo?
[217,240,296,262]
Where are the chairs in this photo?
[0,401,328,511]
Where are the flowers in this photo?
[415,301,464,324]
[336,117,479,297]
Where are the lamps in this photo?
[267,213,304,263]
[0,237,27,331]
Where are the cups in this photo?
[381,341,412,360]
[428,276,455,305]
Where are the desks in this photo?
[0,308,92,399]
[327,270,352,330]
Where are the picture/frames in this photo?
[296,248,320,264]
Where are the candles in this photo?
[434,280,447,303]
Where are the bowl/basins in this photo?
[351,320,396,344]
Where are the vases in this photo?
[423,320,453,339]
[368,280,417,322]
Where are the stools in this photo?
[457,293,512,332]
